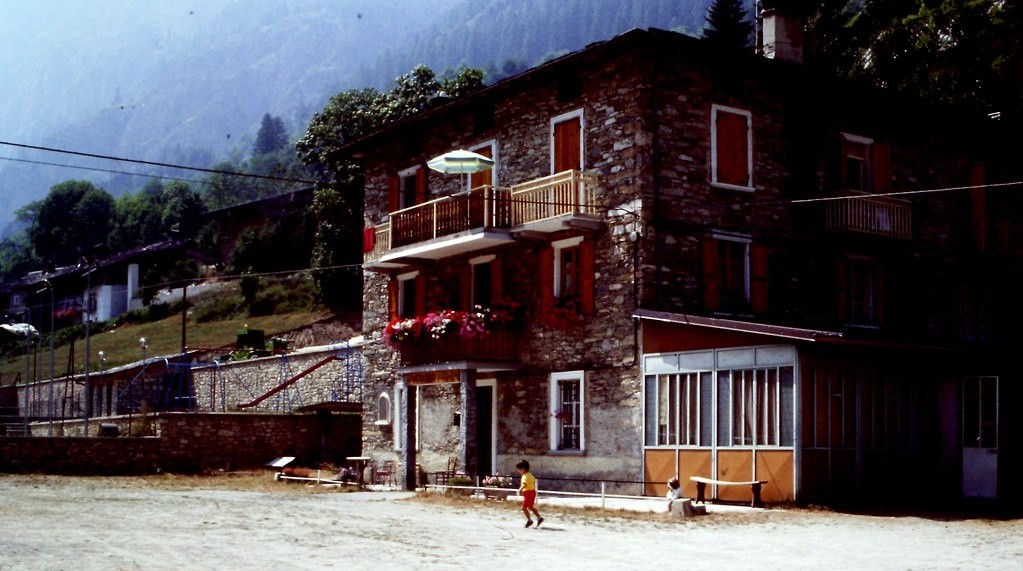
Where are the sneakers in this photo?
[536,517,544,528]
[525,520,533,528]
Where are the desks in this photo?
[346,457,372,491]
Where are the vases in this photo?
[483,472,513,501]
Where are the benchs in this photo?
[689,476,769,509]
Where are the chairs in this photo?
[435,456,458,485]
[373,459,398,487]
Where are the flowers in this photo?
[380,304,491,352]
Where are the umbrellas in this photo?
[427,149,495,192]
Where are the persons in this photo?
[515,460,544,528]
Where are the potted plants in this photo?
[448,477,476,498]
